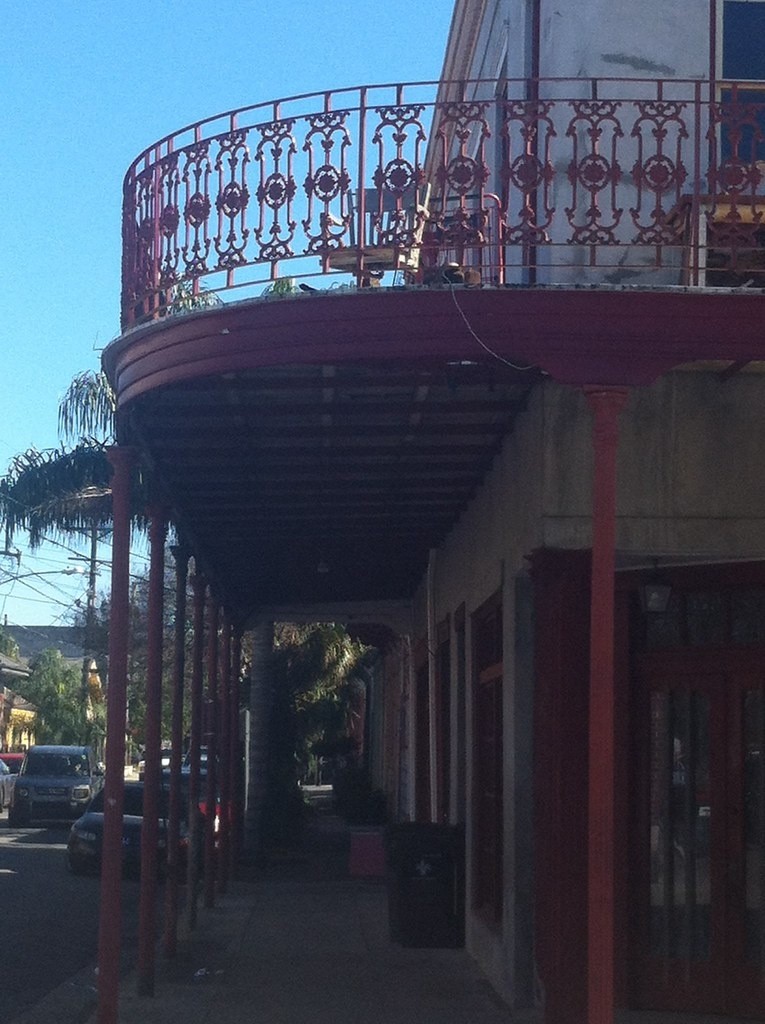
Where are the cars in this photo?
[181,746,220,785]
[66,779,193,882]
[139,747,185,780]
[0,759,12,814]
[9,745,104,826]
